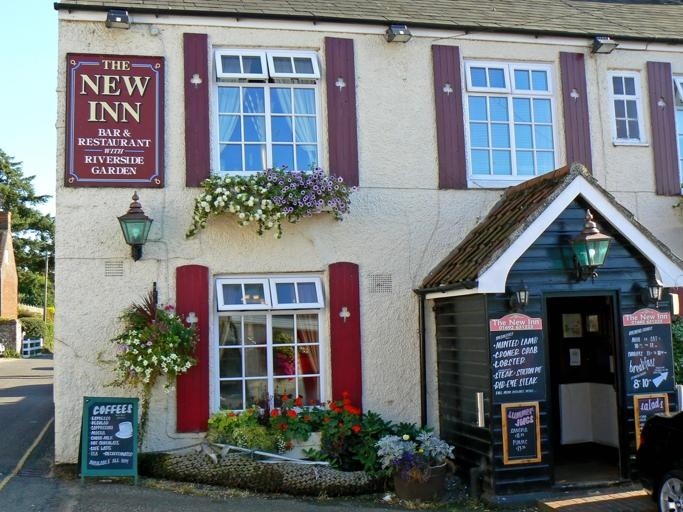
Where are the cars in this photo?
[635,409,683,511]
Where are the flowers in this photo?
[186,162,360,241]
[375,430,454,483]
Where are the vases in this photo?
[393,462,447,501]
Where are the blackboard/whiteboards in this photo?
[623,324,676,451]
[81,396,139,477]
[490,329,547,465]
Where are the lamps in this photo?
[386,25,412,43]
[510,279,530,315]
[118,191,154,261]
[592,37,619,53]
[640,277,663,310]
[106,10,130,30]
[570,209,611,278]
